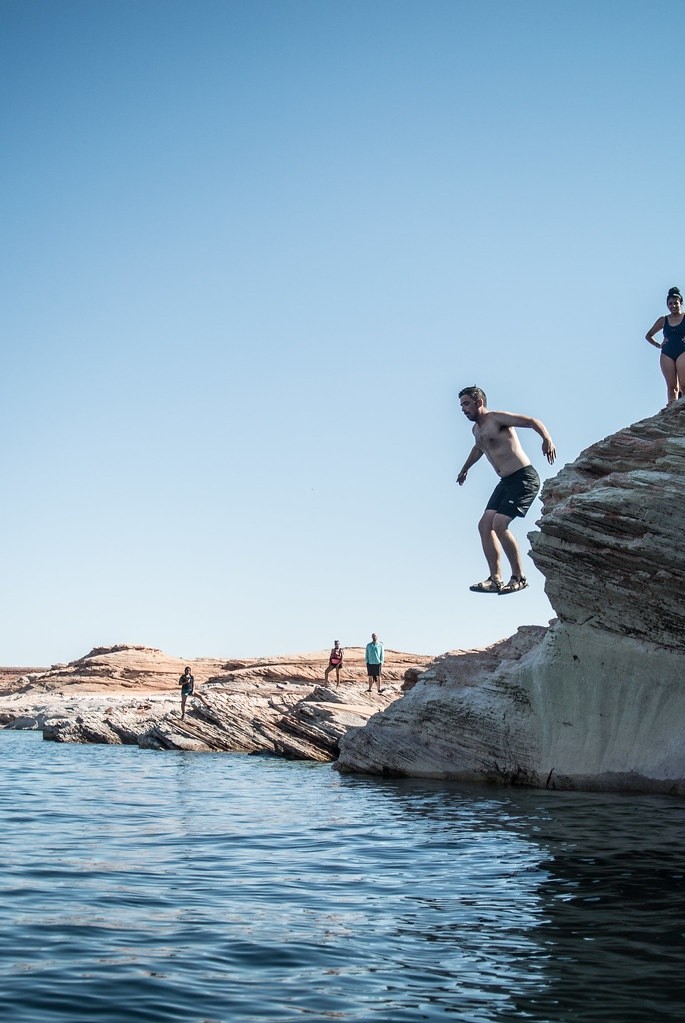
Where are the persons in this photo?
[366,634,384,693]
[179,667,211,721]
[456,387,556,596]
[325,640,343,688]
[645,287,685,404]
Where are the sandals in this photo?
[498,575,528,595]
[469,576,504,592]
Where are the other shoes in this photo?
[182,714,185,718]
[207,705,212,708]
[378,690,382,693]
[367,690,371,692]
